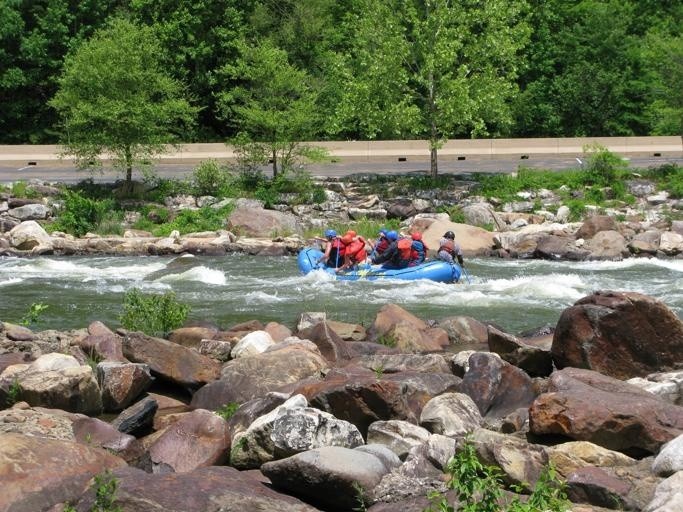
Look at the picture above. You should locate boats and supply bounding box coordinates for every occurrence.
[297,247,461,287]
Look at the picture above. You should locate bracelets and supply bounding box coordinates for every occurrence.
[317,259,320,263]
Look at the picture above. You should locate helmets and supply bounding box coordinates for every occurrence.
[380,228,397,241]
[412,232,421,240]
[325,229,336,236]
[342,231,356,242]
[443,231,455,238]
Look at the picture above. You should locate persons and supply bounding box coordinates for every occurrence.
[367,228,429,270]
[346,229,356,238]
[314,228,345,268]
[334,234,368,274]
[435,230,465,268]
